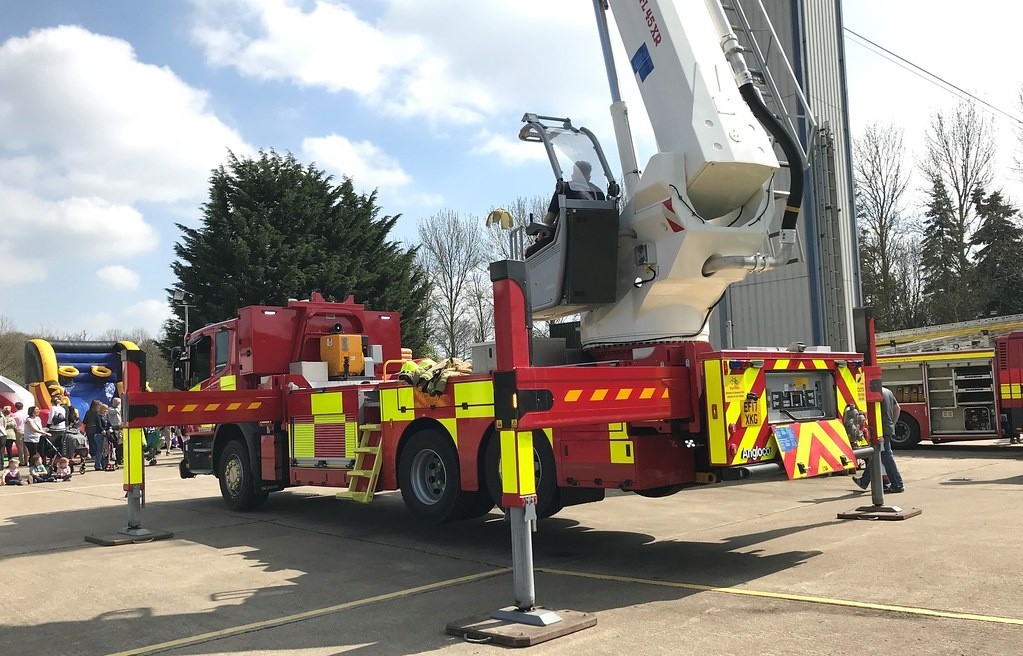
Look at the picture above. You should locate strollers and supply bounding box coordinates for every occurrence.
[44,427,90,475]
[142,426,167,466]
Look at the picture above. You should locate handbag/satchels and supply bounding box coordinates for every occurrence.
[53,406,66,425]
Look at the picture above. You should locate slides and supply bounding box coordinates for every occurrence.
[58,376,122,431]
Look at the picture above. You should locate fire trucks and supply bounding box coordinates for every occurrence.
[876,312,1023,451]
[80,0,924,647]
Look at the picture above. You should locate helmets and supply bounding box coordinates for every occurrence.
[401,361,418,373]
[420,359,436,368]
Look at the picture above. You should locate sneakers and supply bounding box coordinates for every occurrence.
[883,484,904,493]
[852,476,869,490]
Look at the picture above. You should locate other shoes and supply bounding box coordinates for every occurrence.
[46,478,55,482]
[20,481,28,485]
[166,451,170,456]
[63,477,71,482]
[95,467,104,471]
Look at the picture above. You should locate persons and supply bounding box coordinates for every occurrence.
[0,395,206,485]
[853,387,904,493]
[525,162,606,243]
